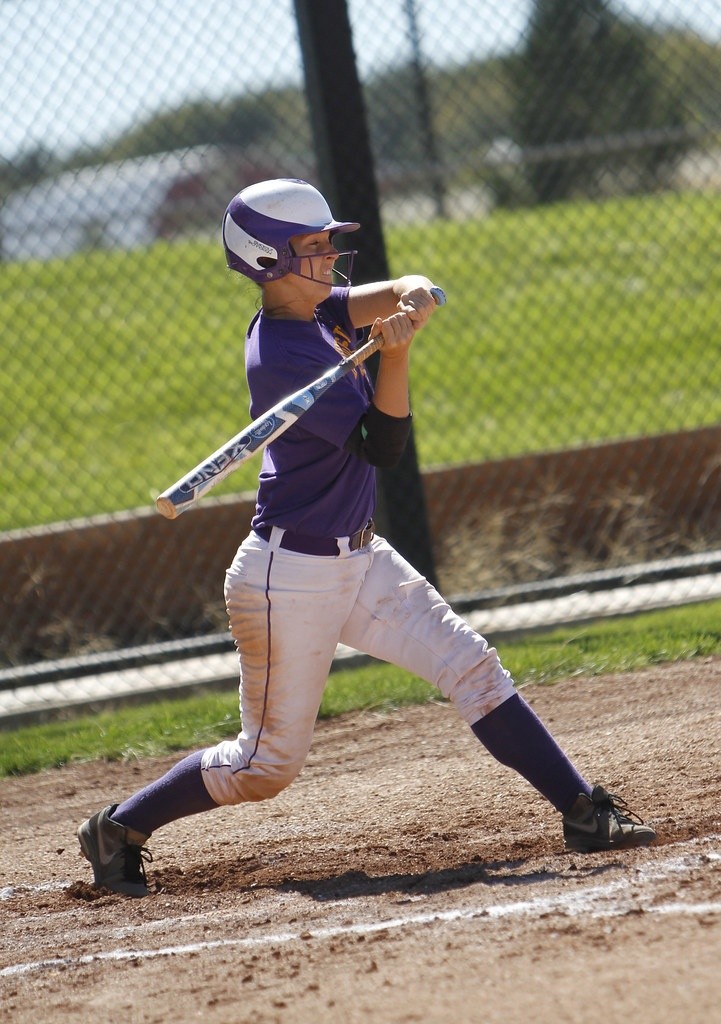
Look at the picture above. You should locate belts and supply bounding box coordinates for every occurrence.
[255,519,374,556]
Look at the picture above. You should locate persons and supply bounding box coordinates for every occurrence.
[80,177,656,893]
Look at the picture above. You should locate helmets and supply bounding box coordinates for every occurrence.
[221,179,358,288]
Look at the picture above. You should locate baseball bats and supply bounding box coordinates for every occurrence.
[152,286,448,522]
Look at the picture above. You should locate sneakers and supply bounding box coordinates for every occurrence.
[561,785,655,854]
[78,804,153,899]
